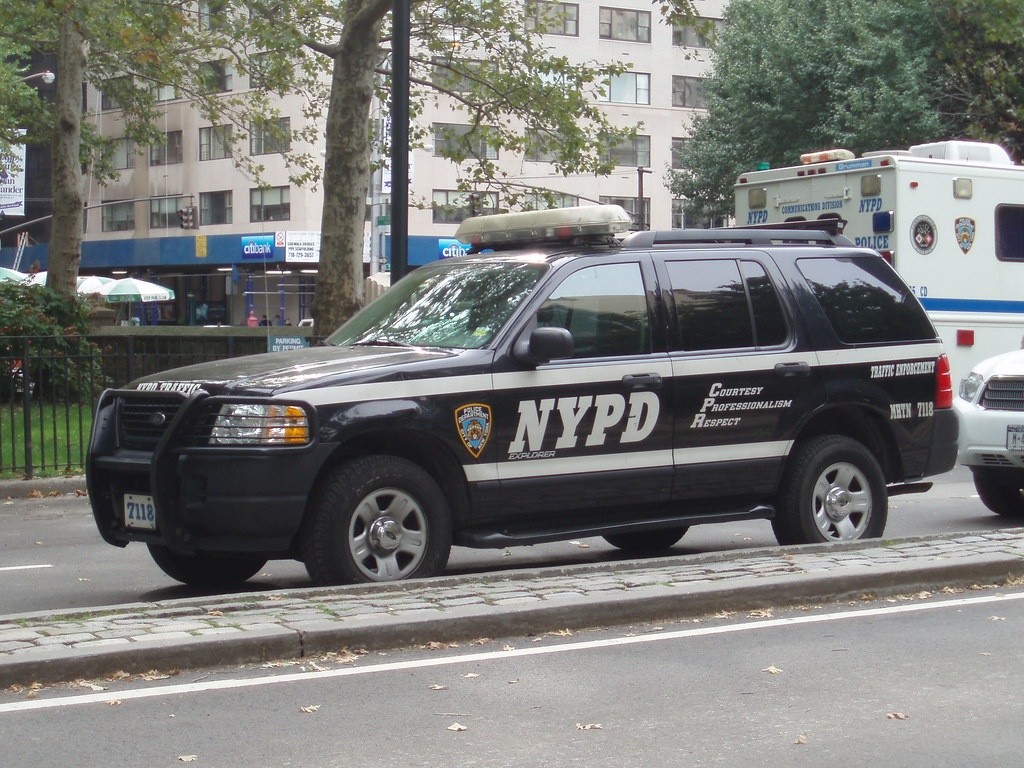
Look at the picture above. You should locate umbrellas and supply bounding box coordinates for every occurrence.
[0,267,28,282]
[17,271,46,286]
[76,275,116,297]
[86,277,175,326]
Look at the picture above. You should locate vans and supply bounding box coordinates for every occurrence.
[731,141,1024,427]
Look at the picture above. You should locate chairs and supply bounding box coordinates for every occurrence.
[567,286,646,359]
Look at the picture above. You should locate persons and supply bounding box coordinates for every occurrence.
[258,315,271,326]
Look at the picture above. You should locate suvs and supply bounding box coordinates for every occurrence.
[85,201,960,592]
